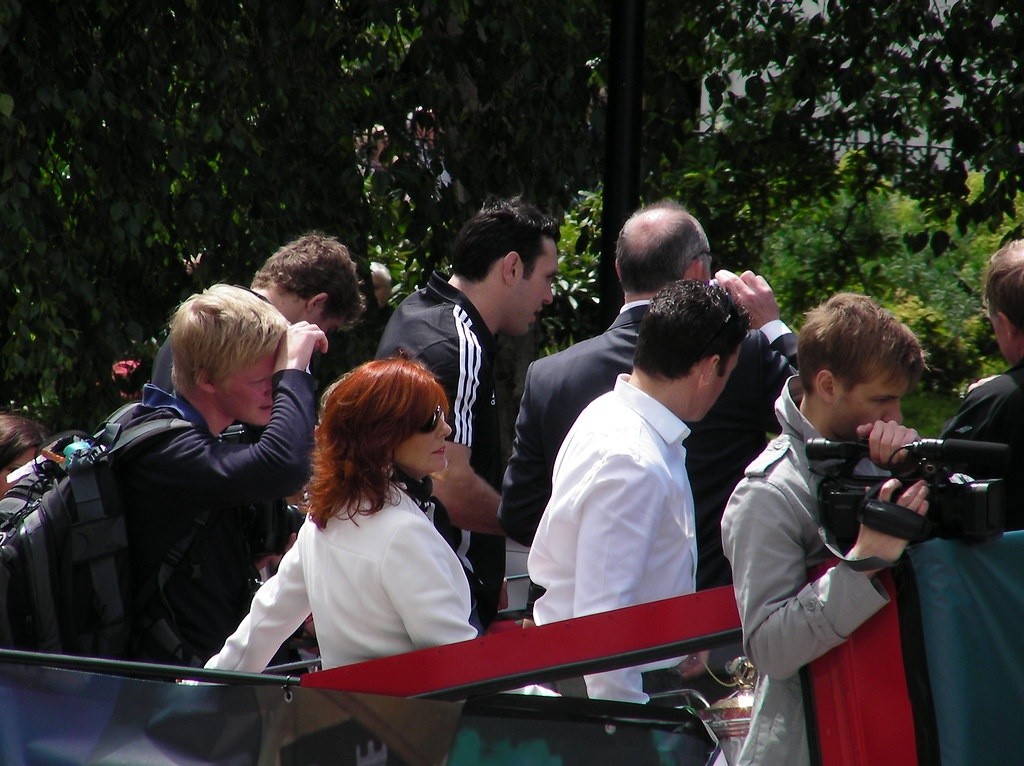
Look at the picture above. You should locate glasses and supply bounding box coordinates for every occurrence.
[417,403,444,434]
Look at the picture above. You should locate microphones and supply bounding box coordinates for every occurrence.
[906,438,1011,465]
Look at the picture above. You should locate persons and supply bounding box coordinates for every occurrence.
[525,280,748,701]
[497,205,798,701]
[721,294,931,766]
[96,285,328,684]
[151,235,389,428]
[0,413,45,501]
[172,357,476,695]
[352,106,457,227]
[936,238,1024,530]
[373,198,558,628]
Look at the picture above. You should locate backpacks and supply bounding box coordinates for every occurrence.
[0,418,211,718]
